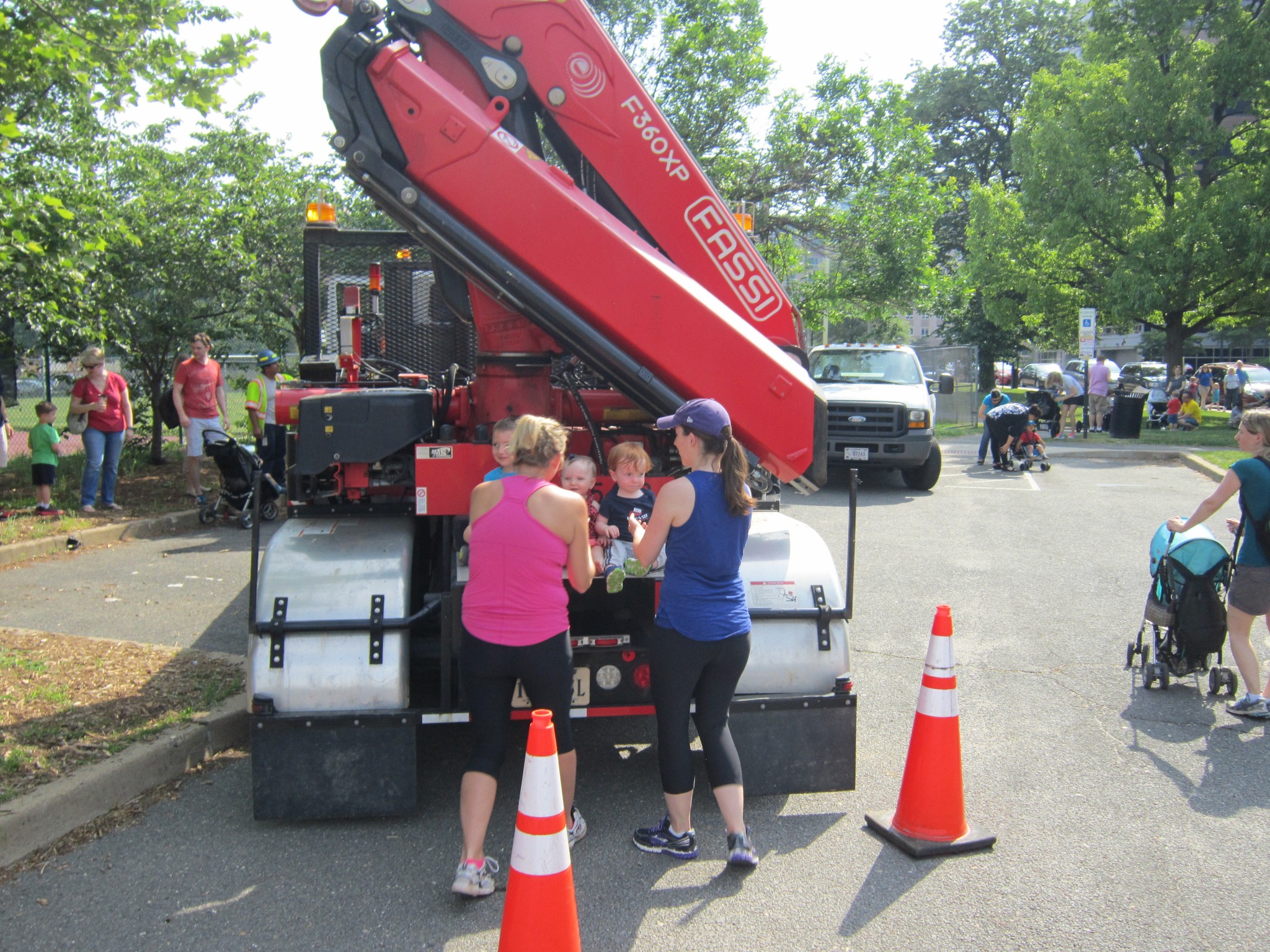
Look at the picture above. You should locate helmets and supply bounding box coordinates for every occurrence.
[255,350,281,366]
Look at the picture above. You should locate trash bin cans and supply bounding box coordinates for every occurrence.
[1109,389,1150,439]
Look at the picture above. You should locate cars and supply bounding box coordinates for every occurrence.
[1017,363,1064,390]
[920,361,968,382]
[993,362,1014,385]
[967,360,980,383]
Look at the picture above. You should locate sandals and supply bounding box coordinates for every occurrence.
[1000,465,1017,472]
[992,461,1000,470]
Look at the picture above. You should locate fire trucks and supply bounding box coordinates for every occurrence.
[201,0,860,822]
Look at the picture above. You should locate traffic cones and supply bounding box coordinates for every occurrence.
[858,606,1001,861]
[500,707,586,952]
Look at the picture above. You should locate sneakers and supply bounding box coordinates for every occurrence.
[450,857,501,896]
[633,813,698,858]
[1225,692,1270,718]
[726,829,759,865]
[566,808,587,850]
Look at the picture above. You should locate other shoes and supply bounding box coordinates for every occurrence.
[1054,433,1066,439]
[274,484,286,494]
[1042,456,1049,461]
[200,485,213,492]
[1200,407,1208,411]
[1030,456,1037,462]
[1089,428,1096,432]
[1068,434,1074,439]
[606,568,624,593]
[623,557,653,577]
[194,495,208,507]
[1096,429,1106,434]
[977,459,984,464]
[0,503,122,521]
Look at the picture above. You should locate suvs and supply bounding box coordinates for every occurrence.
[1117,361,1168,400]
[808,343,954,492]
[1189,362,1270,409]
[1062,358,1121,395]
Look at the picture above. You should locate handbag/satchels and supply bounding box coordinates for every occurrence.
[65,402,90,434]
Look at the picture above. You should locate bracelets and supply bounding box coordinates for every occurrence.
[127,427,134,430]
[4,419,9,423]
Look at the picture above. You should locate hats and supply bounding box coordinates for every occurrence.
[657,398,732,441]
[1026,420,1037,426]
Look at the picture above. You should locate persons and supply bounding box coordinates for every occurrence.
[627,399,758,867]
[1044,355,1117,442]
[555,454,610,573]
[1160,359,1251,431]
[69,348,134,513]
[27,399,65,516]
[976,386,1048,472]
[0,381,14,519]
[484,415,523,483]
[1166,402,1270,722]
[245,349,296,492]
[594,443,666,593]
[173,331,231,505]
[452,413,596,895]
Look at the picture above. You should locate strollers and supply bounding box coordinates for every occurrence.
[1008,432,1052,471]
[199,428,282,530]
[1123,510,1247,697]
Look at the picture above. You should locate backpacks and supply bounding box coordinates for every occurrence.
[1164,556,1231,655]
[157,388,180,429]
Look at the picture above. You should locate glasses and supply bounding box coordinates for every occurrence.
[82,364,98,370]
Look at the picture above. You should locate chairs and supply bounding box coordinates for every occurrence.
[880,365,907,381]
[818,365,842,380]
